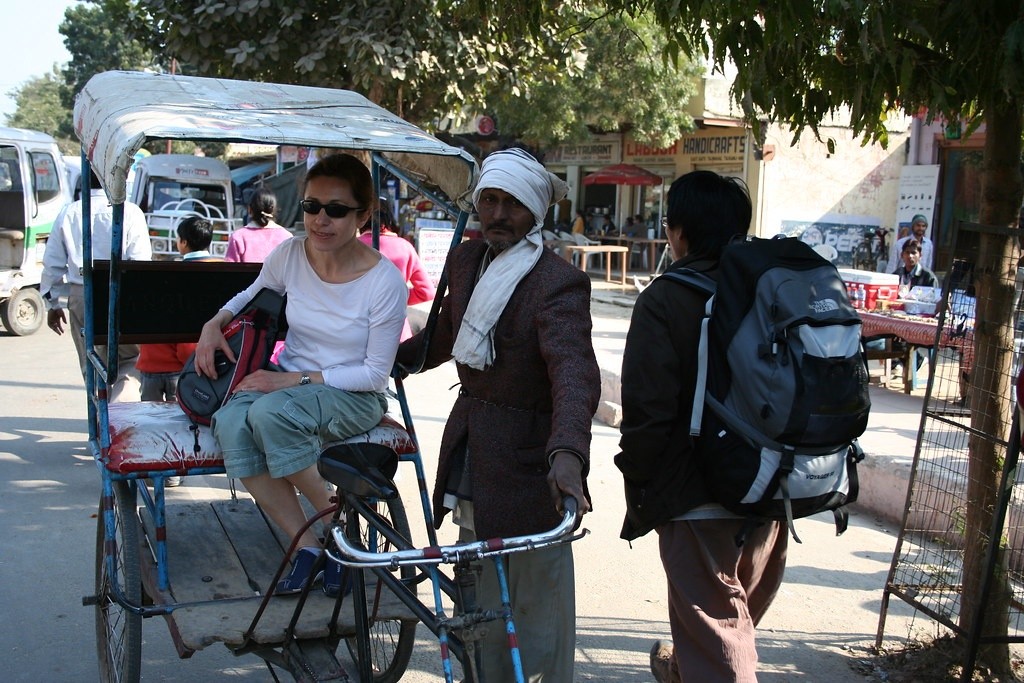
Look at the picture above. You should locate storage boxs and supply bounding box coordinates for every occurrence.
[839,269,899,311]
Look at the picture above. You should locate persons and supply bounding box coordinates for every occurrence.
[886,214,934,274]
[139,218,228,486]
[602,215,615,235]
[391,147,601,683]
[617,215,647,250]
[195,152,408,597]
[544,193,593,237]
[613,171,788,683]
[224,189,293,262]
[40,172,152,401]
[890,239,940,376]
[357,195,435,344]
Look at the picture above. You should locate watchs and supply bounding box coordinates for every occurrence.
[301,371,311,385]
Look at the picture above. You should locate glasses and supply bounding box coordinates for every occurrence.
[661,214,674,226]
[299,200,363,218]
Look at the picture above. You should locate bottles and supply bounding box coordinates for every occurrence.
[845,282,851,304]
[851,283,859,308]
[858,284,866,308]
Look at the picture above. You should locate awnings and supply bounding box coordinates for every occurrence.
[231,159,275,186]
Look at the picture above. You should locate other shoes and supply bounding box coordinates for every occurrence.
[917,352,927,373]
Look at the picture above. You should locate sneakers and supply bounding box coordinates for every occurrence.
[323,546,352,598]
[275,547,325,593]
[650,639,676,683]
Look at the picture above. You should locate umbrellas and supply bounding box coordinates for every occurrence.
[584,161,662,235]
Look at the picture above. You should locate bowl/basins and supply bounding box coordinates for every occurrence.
[602,208,611,214]
[594,208,602,213]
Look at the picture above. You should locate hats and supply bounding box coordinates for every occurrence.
[912,215,928,228]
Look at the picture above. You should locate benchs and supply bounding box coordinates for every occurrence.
[0,190,57,238]
[90,259,417,474]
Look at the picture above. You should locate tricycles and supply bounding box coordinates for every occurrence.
[67,70,593,683]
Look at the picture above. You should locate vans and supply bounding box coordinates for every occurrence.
[0,125,74,337]
[129,150,236,261]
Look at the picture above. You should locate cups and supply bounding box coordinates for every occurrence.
[601,230,604,236]
[647,229,655,239]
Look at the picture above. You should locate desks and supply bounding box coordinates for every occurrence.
[589,236,668,273]
[853,309,976,395]
[566,245,628,284]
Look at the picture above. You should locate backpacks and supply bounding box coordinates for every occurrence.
[653,234,871,546]
[176,287,281,454]
